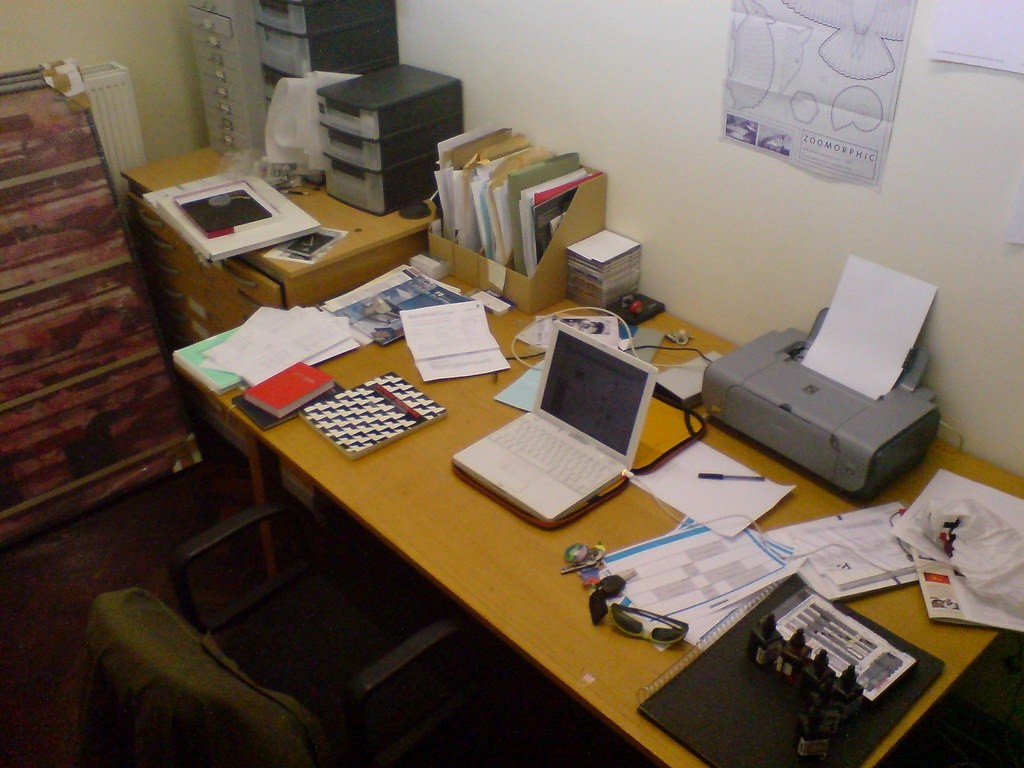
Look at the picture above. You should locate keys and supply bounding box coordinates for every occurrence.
[560,545,640,596]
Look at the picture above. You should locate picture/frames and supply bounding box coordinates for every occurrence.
[0,59,204,547]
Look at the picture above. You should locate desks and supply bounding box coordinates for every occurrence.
[172,256,1024,768]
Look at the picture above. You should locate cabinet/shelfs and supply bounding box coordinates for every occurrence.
[121,148,428,516]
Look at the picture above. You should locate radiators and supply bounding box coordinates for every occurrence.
[80,59,146,206]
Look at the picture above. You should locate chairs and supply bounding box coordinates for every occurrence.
[78,498,478,768]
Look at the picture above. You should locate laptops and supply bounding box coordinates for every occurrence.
[452,320,658,523]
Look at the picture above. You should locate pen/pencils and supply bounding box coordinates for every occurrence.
[697,472,765,481]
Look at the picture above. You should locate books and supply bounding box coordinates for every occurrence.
[639,573,946,768]
[171,262,475,431]
[299,371,446,459]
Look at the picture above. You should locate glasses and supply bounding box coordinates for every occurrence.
[611,603,689,644]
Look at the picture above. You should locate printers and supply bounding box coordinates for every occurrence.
[702,308,941,509]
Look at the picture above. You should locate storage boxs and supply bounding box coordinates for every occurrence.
[434,169,607,315]
[190,0,465,216]
